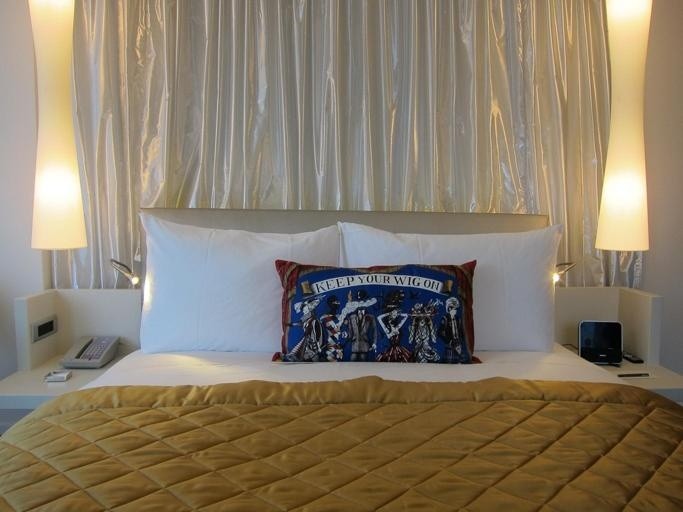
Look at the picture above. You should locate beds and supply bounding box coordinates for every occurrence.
[0,208,683,512]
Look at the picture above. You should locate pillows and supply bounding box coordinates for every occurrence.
[337,221,562,352]
[271,259,484,365]
[137,213,340,355]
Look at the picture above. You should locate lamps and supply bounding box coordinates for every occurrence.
[553,262,576,282]
[595,0,653,251]
[110,259,141,289]
[28,0,87,250]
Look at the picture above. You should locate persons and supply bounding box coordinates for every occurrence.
[287,287,464,363]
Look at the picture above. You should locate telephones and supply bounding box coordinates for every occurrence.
[63,335,120,369]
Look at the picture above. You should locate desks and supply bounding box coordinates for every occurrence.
[555,286,683,406]
[0,289,141,436]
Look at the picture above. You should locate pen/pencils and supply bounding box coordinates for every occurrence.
[618,373,649,377]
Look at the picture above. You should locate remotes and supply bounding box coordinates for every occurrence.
[623,350,644,363]
